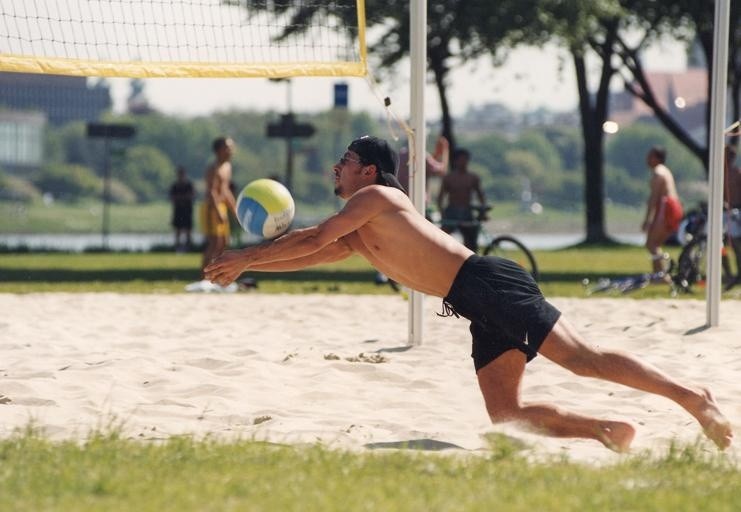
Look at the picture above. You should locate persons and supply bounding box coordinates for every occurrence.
[642,145,683,286]
[168,165,197,249]
[435,148,489,252]
[377,133,451,287]
[203,135,736,456]
[201,133,240,284]
[720,146,741,293]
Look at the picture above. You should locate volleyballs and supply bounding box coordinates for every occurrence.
[236,179,295,239]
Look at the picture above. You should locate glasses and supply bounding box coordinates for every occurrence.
[337,154,360,168]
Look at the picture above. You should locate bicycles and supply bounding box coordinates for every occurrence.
[385,203,543,301]
[577,252,676,296]
[671,204,741,297]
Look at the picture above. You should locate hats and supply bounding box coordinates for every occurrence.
[347,133,407,192]
